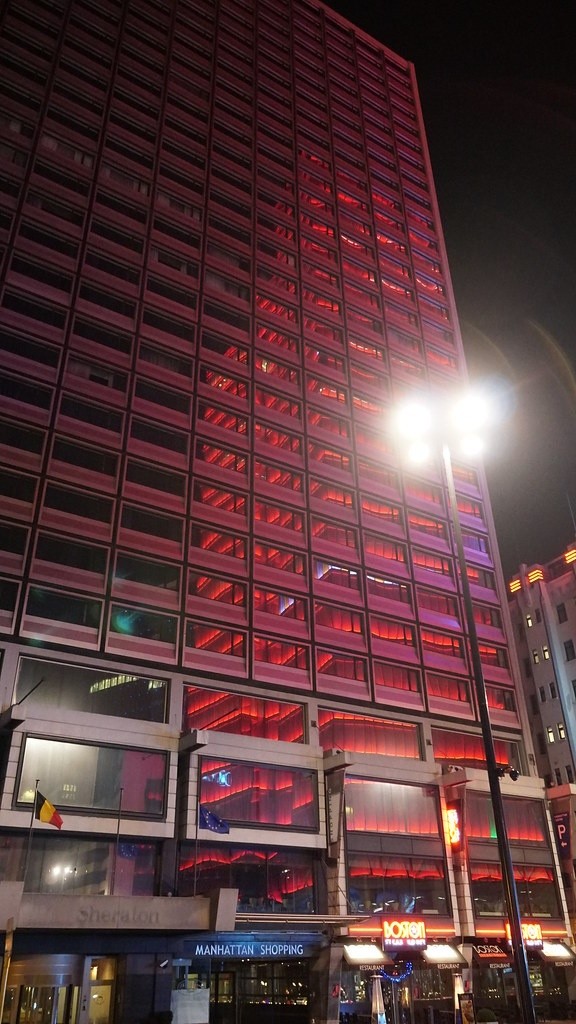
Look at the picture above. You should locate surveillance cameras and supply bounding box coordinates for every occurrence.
[509,771,520,781]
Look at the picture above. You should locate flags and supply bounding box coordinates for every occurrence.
[116,841,138,859]
[32,783,64,830]
[196,801,230,834]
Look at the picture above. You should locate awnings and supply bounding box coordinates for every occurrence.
[536,939,576,966]
[472,943,514,964]
[420,943,468,969]
[344,942,396,971]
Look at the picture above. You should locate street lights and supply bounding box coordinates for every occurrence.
[386,382,539,1023]
